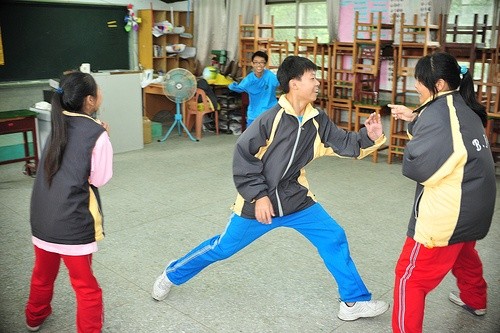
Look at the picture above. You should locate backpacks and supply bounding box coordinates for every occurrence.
[195,77,217,110]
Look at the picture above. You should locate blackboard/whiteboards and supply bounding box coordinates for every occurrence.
[0,0,140,86]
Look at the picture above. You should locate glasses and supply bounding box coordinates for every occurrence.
[252,61,267,66]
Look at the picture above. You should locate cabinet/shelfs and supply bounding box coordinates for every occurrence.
[138,9,195,76]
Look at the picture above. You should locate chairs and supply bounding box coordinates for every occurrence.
[182,88,219,141]
[238,10,500,164]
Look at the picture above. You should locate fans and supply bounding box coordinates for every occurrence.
[157,68,200,143]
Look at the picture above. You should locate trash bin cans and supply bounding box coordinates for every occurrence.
[142,117,152,144]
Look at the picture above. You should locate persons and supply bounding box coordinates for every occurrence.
[387,52,497,333]
[25,72,113,333]
[152,55,390,322]
[228,51,280,128]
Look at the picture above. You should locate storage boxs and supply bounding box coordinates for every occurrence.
[211,50,227,65]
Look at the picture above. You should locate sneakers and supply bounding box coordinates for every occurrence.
[338,298,390,320]
[449,291,486,316]
[152,271,173,301]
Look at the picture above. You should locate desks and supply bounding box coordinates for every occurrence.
[142,75,232,139]
[0,108,41,176]
